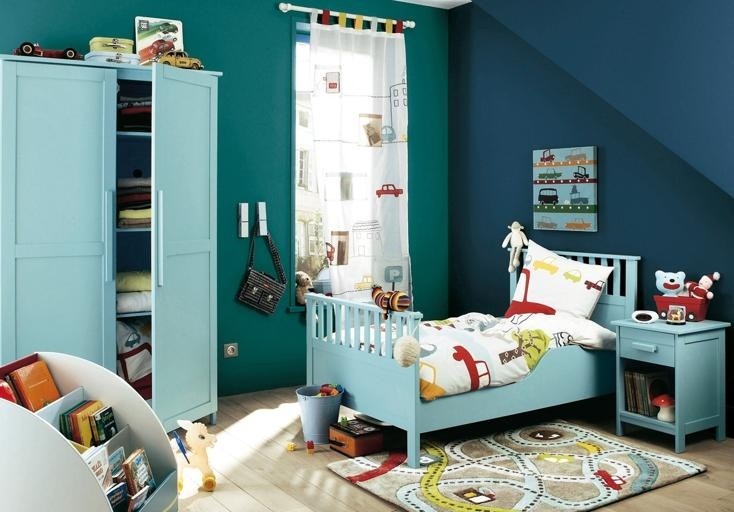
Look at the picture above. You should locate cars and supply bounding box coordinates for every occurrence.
[147,20,178,55]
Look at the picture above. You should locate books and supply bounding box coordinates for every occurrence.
[7,361,157,512]
[624,369,675,417]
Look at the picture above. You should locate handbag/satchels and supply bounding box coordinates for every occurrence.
[237,267,284,313]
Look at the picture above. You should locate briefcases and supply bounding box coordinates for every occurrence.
[84,37,140,65]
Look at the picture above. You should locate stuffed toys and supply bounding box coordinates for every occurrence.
[296,270,317,305]
[369,283,411,319]
[502,221,529,273]
[685,271,720,300]
[655,270,686,297]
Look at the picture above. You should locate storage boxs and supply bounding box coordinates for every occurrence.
[327,418,384,458]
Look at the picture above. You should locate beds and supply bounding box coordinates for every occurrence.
[303,247,641,468]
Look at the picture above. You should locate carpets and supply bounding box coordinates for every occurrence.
[325,416,708,512]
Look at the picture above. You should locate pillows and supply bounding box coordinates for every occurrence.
[504,239,615,319]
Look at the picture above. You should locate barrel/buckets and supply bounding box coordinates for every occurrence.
[297,385,346,444]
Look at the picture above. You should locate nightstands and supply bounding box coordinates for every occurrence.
[609,318,731,453]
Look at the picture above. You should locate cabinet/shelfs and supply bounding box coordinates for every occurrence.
[0,352,177,512]
[0,54,223,435]
